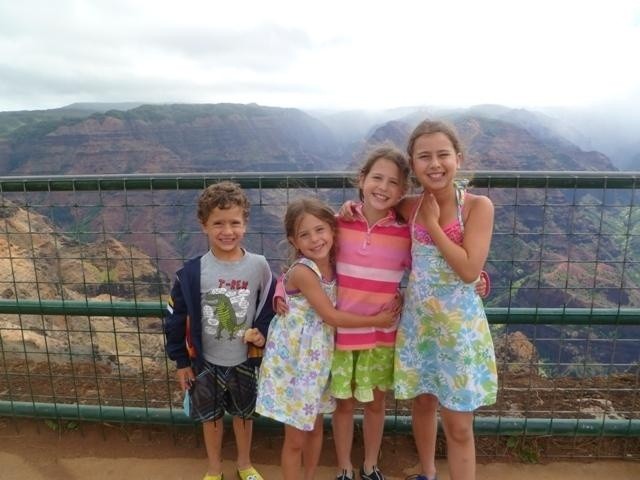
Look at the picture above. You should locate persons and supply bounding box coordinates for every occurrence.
[161,178,279,480]
[338,117,501,480]
[270,142,492,480]
[251,195,404,480]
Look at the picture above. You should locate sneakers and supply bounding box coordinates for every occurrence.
[204,471,224,480]
[358,464,385,480]
[236,465,264,480]
[334,468,355,480]
